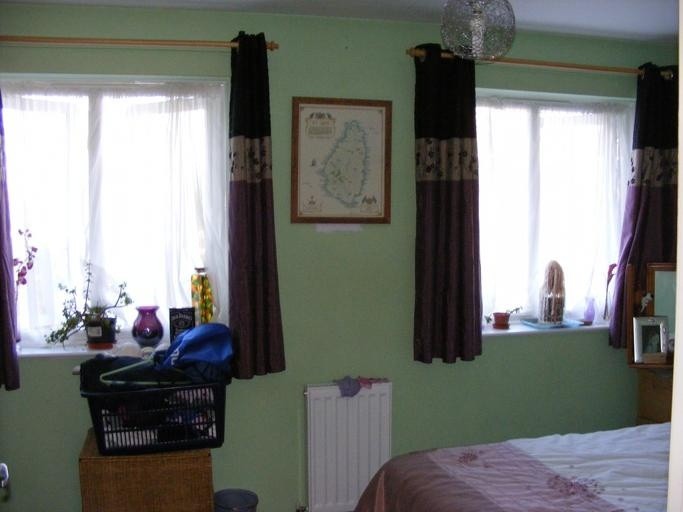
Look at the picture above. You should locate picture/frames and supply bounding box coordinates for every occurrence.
[647,262,676,362]
[290,95,393,224]
[633,315,669,364]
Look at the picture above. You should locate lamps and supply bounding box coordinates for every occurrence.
[438,0,516,60]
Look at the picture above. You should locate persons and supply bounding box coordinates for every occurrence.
[643,328,660,353]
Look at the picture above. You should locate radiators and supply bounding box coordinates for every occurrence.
[304,379,392,511]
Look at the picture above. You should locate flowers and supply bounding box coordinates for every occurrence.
[10,228,38,342]
[44,259,132,350]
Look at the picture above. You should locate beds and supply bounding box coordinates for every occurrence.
[353,421,671,512]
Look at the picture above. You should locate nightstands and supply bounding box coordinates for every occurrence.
[629,364,672,426]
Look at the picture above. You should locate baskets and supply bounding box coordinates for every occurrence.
[79,361,234,458]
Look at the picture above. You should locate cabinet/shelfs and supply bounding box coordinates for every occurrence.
[78,426,215,512]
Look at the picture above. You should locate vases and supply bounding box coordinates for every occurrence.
[131,306,164,349]
[82,318,117,343]
[14,321,22,354]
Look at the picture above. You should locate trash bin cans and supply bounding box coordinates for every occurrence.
[214,488,260,512]
[0,463,10,503]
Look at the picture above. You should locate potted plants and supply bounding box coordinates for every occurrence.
[484,305,522,329]
